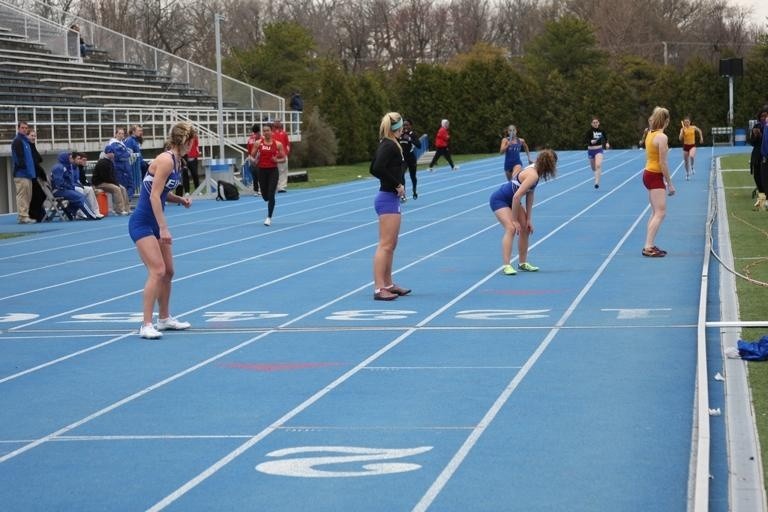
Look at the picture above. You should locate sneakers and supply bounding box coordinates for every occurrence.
[516,263,540,272]
[111,207,128,216]
[17,216,37,224]
[138,321,163,340]
[502,264,517,276]
[278,189,287,193]
[156,316,192,331]
[125,210,134,215]
[263,217,272,226]
[373,287,399,301]
[690,167,696,175]
[686,174,691,181]
[95,212,105,219]
[76,210,88,219]
[642,245,667,258]
[254,191,262,197]
[385,284,412,297]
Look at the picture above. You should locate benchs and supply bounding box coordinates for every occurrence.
[0,26,269,140]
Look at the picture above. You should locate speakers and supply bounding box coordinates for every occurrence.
[719,58,744,76]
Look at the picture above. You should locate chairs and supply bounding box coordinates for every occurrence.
[36,176,79,222]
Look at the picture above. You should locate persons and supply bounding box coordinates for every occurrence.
[638,114,654,146]
[501,125,535,182]
[748,113,768,210]
[128,122,196,339]
[427,119,459,173]
[10,119,291,227]
[639,106,676,257]
[678,117,703,180]
[490,148,558,274]
[397,118,419,201]
[585,118,609,191]
[370,112,412,301]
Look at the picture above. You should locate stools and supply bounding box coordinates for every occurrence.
[95,188,109,217]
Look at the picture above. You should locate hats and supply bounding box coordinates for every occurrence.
[104,145,115,153]
